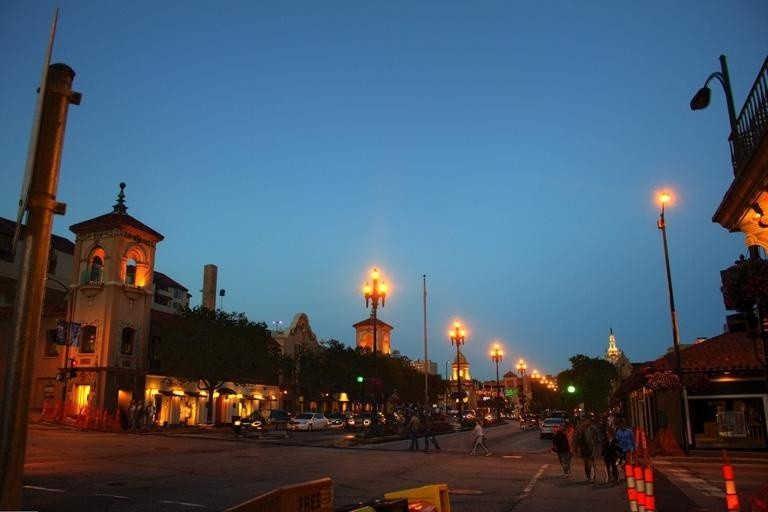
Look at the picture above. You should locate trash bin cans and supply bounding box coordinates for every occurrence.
[233,415,240,429]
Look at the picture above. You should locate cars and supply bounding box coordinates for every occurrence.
[445,408,571,441]
[291,413,329,432]
[326,414,346,431]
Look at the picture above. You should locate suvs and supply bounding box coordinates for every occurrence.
[348,411,386,432]
[233,410,293,441]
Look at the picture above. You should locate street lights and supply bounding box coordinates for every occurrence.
[272,319,282,333]
[653,189,692,458]
[686,50,766,366]
[44,276,74,402]
[362,269,387,437]
[448,319,465,424]
[516,359,558,419]
[491,343,503,424]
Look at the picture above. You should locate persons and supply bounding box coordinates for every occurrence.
[518,407,636,485]
[406,412,492,457]
[128,400,157,433]
